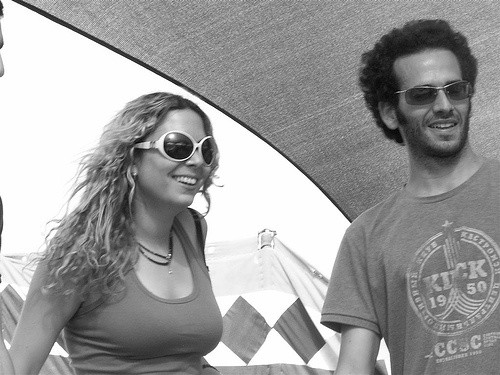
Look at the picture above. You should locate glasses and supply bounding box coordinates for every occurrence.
[133,132,215,166]
[394,81,472,107]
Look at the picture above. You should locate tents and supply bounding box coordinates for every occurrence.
[0,228,391,375]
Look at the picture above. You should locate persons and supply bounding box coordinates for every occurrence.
[320,19,500,375]
[0,0,14,375]
[9,92,223,375]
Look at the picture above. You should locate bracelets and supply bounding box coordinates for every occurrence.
[201,365,220,372]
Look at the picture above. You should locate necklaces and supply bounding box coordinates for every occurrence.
[135,229,174,274]
